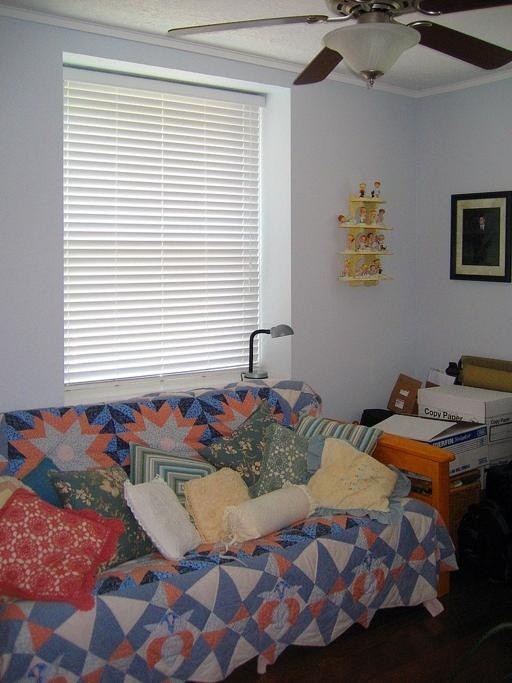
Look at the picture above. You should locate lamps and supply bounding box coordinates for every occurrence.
[321,9,421,88]
[243,325,294,379]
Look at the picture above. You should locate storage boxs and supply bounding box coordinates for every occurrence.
[457,356,512,392]
[418,385,512,462]
[387,374,438,416]
[373,412,488,481]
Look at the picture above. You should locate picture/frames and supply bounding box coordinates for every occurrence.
[450,191,511,283]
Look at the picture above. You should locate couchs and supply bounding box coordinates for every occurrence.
[0,380,454,683]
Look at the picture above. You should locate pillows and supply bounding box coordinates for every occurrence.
[227,480,313,538]
[48,465,158,574]
[123,474,205,563]
[250,424,310,500]
[129,441,217,528]
[295,415,384,458]
[0,487,125,612]
[198,399,285,497]
[184,467,251,545]
[303,435,412,525]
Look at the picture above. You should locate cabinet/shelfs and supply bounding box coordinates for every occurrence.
[336,197,395,287]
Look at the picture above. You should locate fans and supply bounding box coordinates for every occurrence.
[166,1,509,85]
